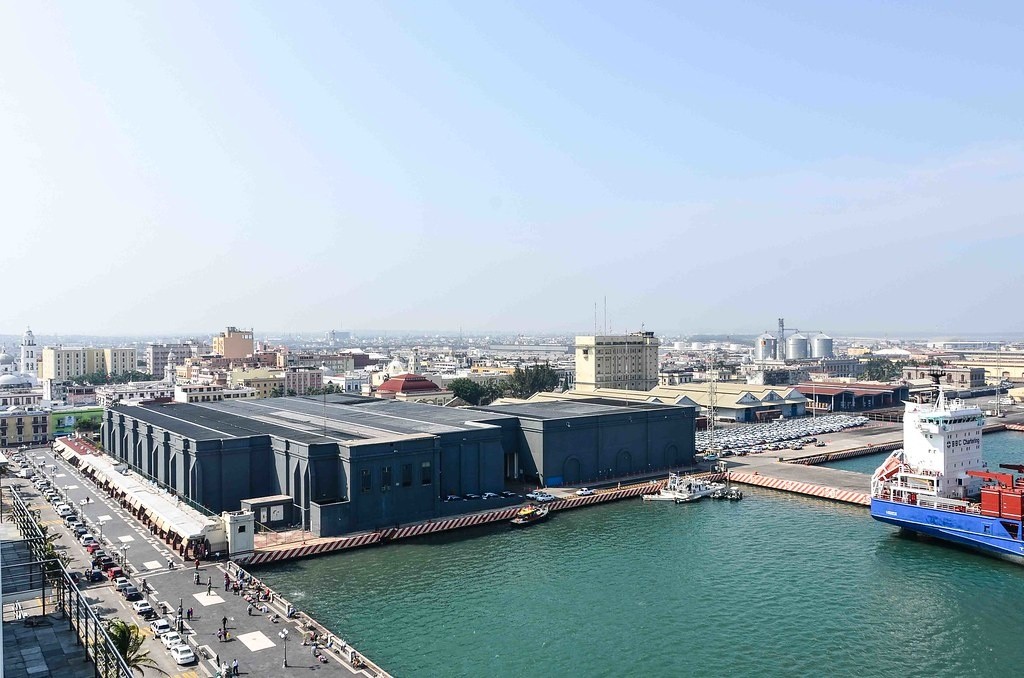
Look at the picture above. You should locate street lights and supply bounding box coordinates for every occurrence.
[62,484,69,504]
[96,521,106,541]
[39,462,45,475]
[49,472,56,487]
[120,543,131,567]
[29,452,36,466]
[278,628,288,668]
[78,502,86,520]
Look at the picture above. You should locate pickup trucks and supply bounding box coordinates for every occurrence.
[64,570,80,588]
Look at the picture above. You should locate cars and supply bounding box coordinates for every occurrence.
[161,632,182,651]
[695,415,869,457]
[171,644,195,665]
[526,490,540,501]
[695,414,870,462]
[133,599,152,615]
[89,570,103,583]
[576,488,593,496]
[447,491,516,501]
[13,452,140,601]
[537,494,555,503]
[151,618,171,640]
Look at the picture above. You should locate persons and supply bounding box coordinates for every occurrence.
[178,605,194,621]
[224,568,298,618]
[167,546,220,569]
[39,450,90,505]
[216,615,231,642]
[300,616,362,668]
[193,571,212,596]
[221,659,240,677]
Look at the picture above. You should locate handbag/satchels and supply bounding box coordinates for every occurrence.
[218,634,222,636]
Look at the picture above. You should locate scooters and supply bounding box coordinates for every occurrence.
[144,611,160,622]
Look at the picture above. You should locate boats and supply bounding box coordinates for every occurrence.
[641,473,726,504]
[728,487,743,502]
[869,448,1024,565]
[508,505,548,528]
